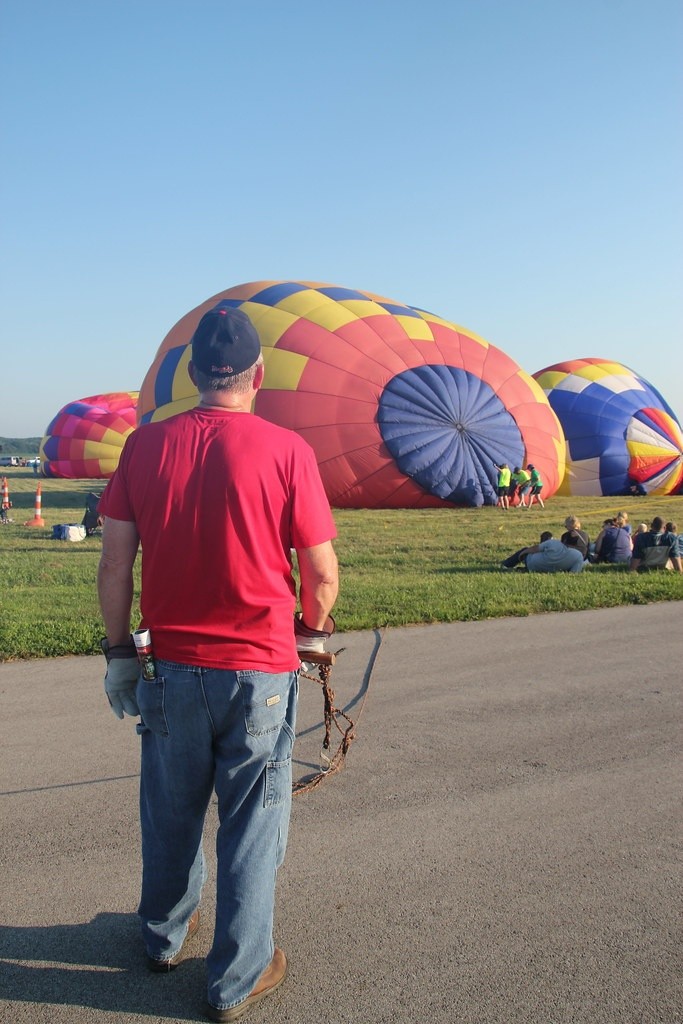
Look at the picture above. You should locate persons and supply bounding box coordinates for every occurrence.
[98,305,338,1024]
[501,510,683,575]
[493,463,544,510]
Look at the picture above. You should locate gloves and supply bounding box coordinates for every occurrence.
[100,638,142,719]
[294,611,336,673]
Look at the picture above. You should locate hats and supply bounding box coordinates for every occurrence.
[193,306,260,377]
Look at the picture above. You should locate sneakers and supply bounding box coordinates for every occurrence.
[209,946,288,1022]
[146,909,200,972]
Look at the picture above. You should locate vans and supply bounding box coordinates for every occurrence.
[0,455,22,467]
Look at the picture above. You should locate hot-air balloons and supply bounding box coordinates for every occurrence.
[136,280,568,510]
[529,356,683,497]
[38,390,139,479]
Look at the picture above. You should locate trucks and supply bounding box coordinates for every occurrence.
[25,456,41,468]
